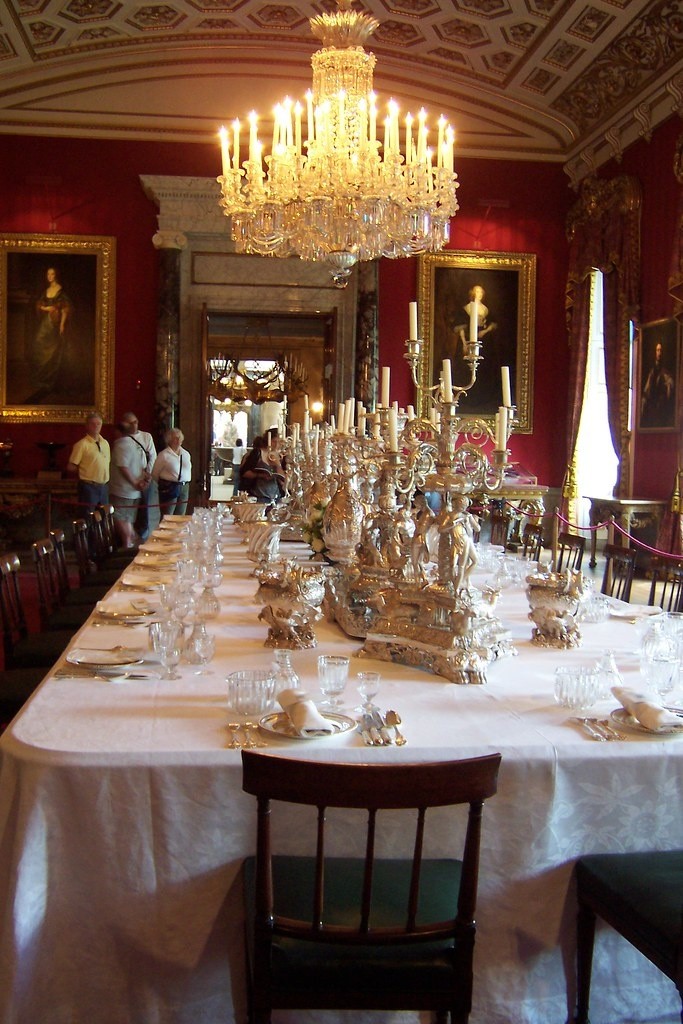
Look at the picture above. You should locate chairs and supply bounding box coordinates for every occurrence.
[557,531,587,573]
[568,849,683,1024]
[601,544,638,603]
[488,513,514,552]
[521,523,544,561]
[229,745,502,1024]
[0,503,137,727]
[645,553,683,612]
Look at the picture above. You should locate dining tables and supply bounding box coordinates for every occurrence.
[0,504,683,1024]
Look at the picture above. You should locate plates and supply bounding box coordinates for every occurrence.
[610,706,683,734]
[123,514,188,589]
[95,599,156,619]
[66,647,144,667]
[258,711,358,740]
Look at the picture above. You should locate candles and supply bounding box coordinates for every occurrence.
[267,298,519,471]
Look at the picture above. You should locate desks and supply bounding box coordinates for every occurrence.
[457,482,550,554]
[580,494,669,573]
[0,478,81,551]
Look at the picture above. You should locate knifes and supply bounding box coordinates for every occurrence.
[56,669,149,677]
[354,710,392,747]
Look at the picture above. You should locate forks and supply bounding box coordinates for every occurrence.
[226,721,267,749]
[570,716,624,742]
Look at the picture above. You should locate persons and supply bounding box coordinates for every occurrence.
[239,428,292,502]
[232,438,246,496]
[67,414,111,512]
[108,412,158,549]
[410,494,481,600]
[151,428,192,522]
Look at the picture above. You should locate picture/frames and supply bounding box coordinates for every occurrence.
[416,249,540,437]
[635,314,681,433]
[0,232,120,426]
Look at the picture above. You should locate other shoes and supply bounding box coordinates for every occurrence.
[118,541,133,553]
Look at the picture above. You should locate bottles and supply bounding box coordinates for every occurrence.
[270,649,301,711]
[638,619,674,683]
[593,650,624,698]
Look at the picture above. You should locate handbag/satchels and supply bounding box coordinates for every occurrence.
[238,449,260,492]
[158,483,179,503]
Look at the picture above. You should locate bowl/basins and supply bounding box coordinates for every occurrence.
[228,670,277,714]
[555,664,598,709]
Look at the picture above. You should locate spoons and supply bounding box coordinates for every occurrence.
[58,672,132,682]
[385,710,407,746]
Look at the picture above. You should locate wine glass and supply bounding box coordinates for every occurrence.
[471,539,610,623]
[660,610,683,662]
[318,656,349,714]
[354,672,379,712]
[151,499,288,682]
[650,657,680,708]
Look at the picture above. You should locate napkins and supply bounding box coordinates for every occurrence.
[277,688,335,737]
[612,683,683,732]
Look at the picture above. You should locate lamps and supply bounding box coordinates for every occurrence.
[217,0,462,287]
[209,317,306,406]
